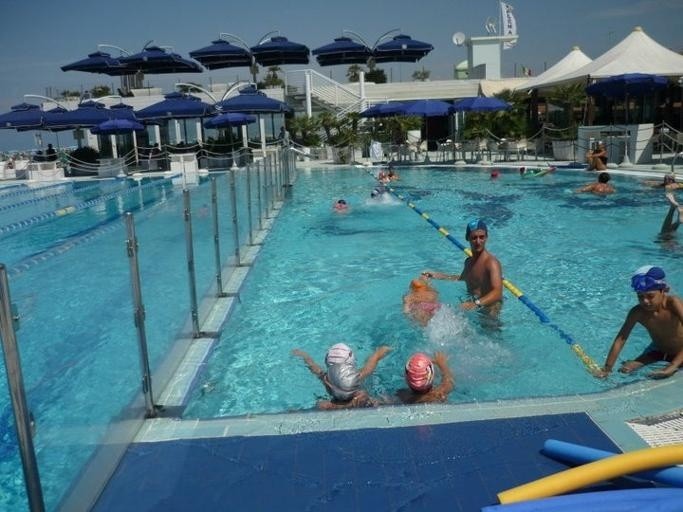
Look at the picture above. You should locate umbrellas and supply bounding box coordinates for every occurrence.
[1,87,292,164]
[514,25,683,125]
[355,96,510,142]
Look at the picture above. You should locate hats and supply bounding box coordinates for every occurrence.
[630,265,670,292]
[323,343,360,400]
[466,218,487,236]
[404,352,434,392]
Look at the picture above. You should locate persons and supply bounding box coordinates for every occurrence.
[577,172,683,378]
[577,141,615,171]
[333,198,349,213]
[520,166,556,179]
[491,169,500,178]
[291,343,454,409]
[403,218,503,328]
[379,169,400,182]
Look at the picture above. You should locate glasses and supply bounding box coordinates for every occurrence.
[631,275,666,290]
